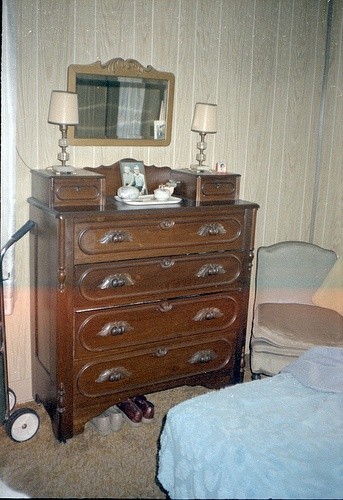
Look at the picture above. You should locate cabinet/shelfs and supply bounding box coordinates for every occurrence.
[27,158,260,443]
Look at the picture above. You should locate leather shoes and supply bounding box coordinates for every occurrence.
[115,398,142,423]
[133,397,154,420]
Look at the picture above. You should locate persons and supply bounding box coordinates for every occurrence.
[133,166,147,195]
[123,166,136,187]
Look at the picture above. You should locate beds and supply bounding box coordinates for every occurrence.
[158,347,343,500]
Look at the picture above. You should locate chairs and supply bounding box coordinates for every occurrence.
[251,242,343,380]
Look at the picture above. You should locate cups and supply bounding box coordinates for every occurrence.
[163,187,174,195]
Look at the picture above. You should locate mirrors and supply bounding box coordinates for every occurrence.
[67,57,175,146]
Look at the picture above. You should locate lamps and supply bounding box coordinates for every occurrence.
[191,103,218,173]
[48,90,79,176]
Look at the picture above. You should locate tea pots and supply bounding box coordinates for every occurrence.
[154,184,170,200]
